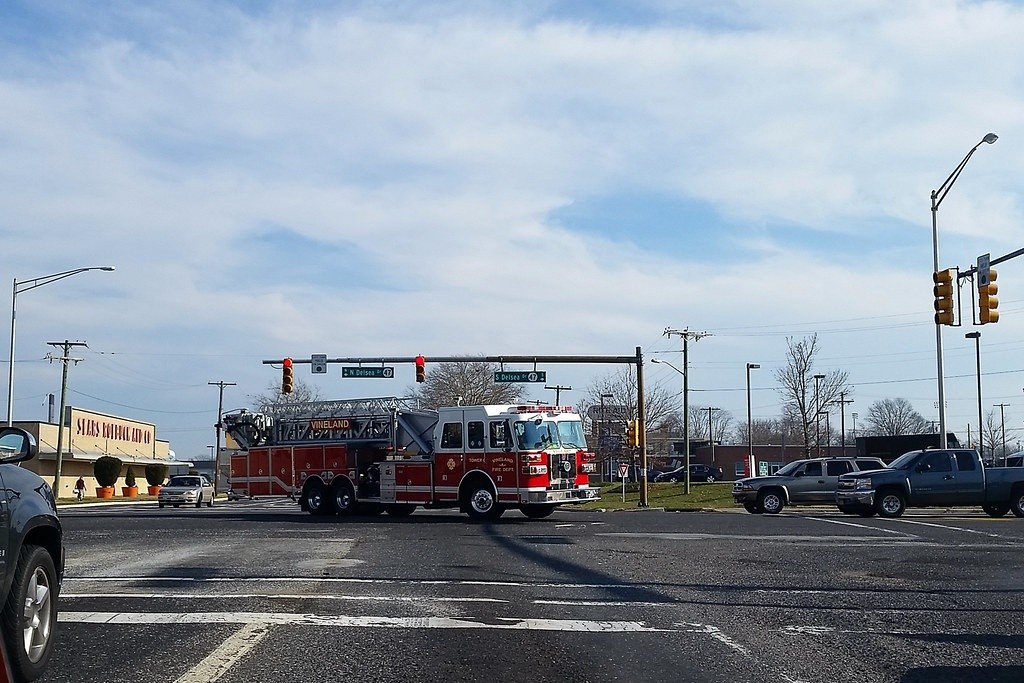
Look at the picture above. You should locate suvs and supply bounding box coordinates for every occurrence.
[732,456,888,514]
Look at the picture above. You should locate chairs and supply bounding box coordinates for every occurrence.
[189,480,196,486]
[176,481,184,485]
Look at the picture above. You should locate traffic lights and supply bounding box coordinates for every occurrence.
[933,270,954,326]
[978,269,999,323]
[415,356,425,382]
[282,357,294,394]
[625,420,639,447]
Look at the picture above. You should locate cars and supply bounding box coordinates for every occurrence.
[158,476,216,508]
[653,464,723,483]
[0,425,66,683]
[228,487,250,502]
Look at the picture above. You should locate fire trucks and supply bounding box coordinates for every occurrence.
[214,394,602,524]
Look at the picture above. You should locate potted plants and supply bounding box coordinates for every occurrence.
[145,463,167,496]
[122,466,138,496]
[94,456,123,499]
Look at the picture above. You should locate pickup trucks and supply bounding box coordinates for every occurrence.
[834,446,1024,519]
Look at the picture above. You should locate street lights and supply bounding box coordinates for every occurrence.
[651,358,689,496]
[965,330,984,459]
[818,411,830,456]
[931,131,999,450]
[206,445,214,461]
[600,393,613,483]
[814,374,825,457]
[7,265,116,429]
[746,362,760,478]
